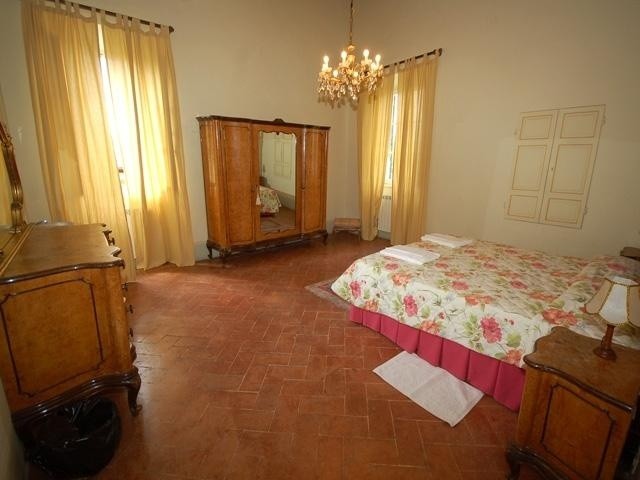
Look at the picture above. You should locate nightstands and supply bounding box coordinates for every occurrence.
[504,326,640,480]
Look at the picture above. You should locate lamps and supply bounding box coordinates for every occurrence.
[314,0,384,103]
[585,276,640,362]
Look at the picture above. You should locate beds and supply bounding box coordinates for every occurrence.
[259,185,282,217]
[330,232,640,412]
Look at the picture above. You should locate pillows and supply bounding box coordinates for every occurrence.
[537,256,640,348]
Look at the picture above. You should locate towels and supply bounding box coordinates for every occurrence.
[379,233,475,265]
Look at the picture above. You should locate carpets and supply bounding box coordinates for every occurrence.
[304,274,351,318]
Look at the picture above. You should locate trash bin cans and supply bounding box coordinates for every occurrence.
[22,397,119,480]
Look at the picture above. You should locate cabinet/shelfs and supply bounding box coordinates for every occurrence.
[1,222,144,464]
[195,115,331,264]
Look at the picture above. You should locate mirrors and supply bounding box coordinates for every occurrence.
[1,120,26,277]
[257,129,298,235]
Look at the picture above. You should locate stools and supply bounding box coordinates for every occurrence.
[333,217,361,246]
